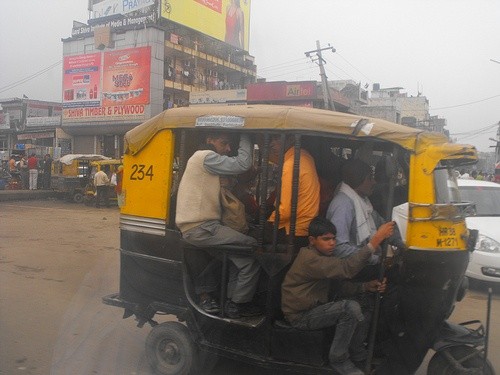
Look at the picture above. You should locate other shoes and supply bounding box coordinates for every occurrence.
[224,302,263,318]
[353,361,376,375]
[329,359,365,375]
[196,292,222,313]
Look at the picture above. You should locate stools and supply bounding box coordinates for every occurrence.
[270,319,334,366]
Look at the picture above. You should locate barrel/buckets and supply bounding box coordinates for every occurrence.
[73,177,82,189]
[64,178,73,190]
[0,177,7,189]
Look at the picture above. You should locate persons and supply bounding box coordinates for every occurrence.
[10,155,27,189]
[27,153,39,191]
[175,134,266,319]
[225,0,244,50]
[231,134,398,359]
[281,217,396,375]
[94,167,112,209]
[455,167,493,181]
[40,154,51,189]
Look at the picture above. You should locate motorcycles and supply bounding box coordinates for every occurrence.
[50,154,122,205]
[103,104,494,373]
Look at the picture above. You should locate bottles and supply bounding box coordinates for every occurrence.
[77,88,86,100]
[90,89,93,99]
[94,84,97,98]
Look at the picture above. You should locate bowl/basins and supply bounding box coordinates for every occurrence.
[10,183,21,191]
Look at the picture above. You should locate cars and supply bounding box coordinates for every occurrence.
[391,176,500,283]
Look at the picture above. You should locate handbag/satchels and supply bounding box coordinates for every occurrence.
[221,186,249,234]
[111,171,118,186]
